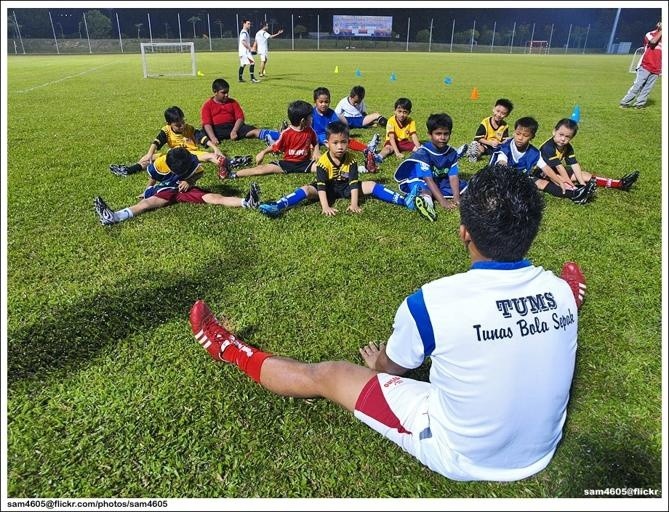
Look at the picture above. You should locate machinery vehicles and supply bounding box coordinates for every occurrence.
[333,21,355,36]
[358,23,368,34]
[371,23,391,36]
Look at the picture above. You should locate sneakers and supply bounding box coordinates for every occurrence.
[364,134,383,173]
[403,184,437,222]
[571,176,596,204]
[244,182,279,219]
[457,141,478,162]
[265,134,283,156]
[94,196,115,227]
[620,171,639,190]
[218,156,253,179]
[190,300,236,362]
[110,164,130,176]
[560,262,586,307]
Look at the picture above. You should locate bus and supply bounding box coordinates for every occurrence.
[526,40,548,48]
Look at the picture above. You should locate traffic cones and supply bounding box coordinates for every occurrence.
[471,86,479,99]
[443,76,453,84]
[390,71,397,80]
[334,65,340,73]
[355,67,361,76]
[571,104,581,123]
[197,70,205,77]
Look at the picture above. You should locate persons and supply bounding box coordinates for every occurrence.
[228,100,381,179]
[538,119,640,192]
[251,22,284,78]
[188,165,584,482]
[257,122,421,218]
[335,86,388,130]
[408,114,470,222]
[194,80,288,147]
[238,20,258,83]
[311,88,380,156]
[108,106,253,177]
[489,118,596,205]
[618,18,663,108]
[364,98,468,163]
[466,98,514,165]
[92,147,261,226]
